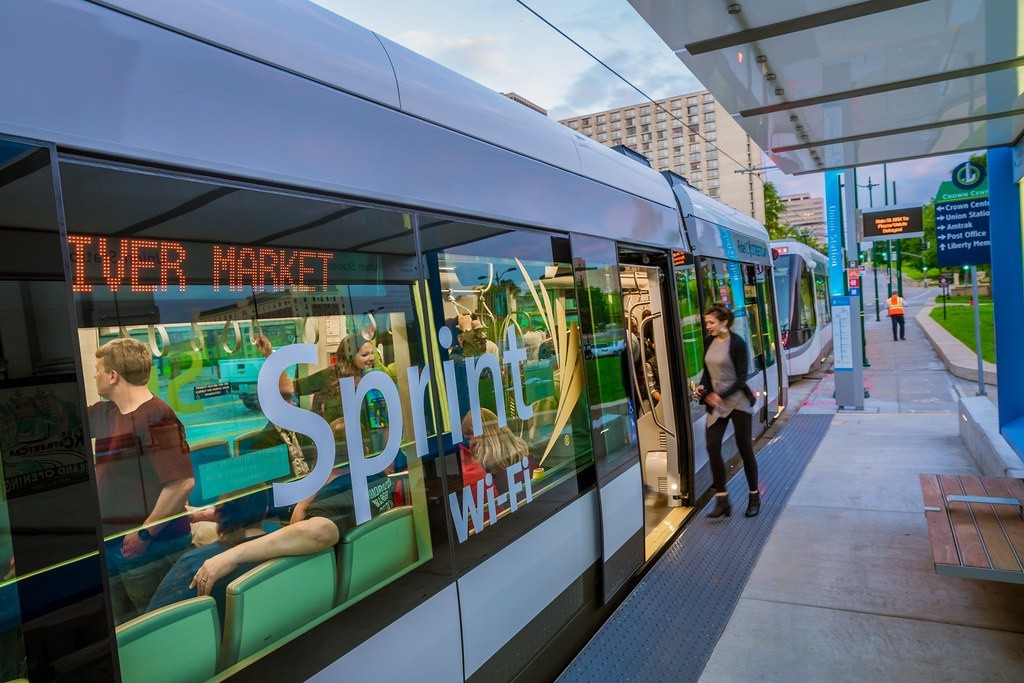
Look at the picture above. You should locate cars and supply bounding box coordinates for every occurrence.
[589,342,612,358]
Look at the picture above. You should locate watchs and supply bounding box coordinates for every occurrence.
[137,529,155,541]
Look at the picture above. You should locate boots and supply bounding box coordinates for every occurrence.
[745,491,761,516]
[705,494,731,517]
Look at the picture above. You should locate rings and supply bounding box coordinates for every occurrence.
[693,391,696,395]
[202,578,207,582]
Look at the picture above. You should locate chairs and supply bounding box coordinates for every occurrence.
[114,596,225,683]
[224,543,339,669]
[337,504,417,603]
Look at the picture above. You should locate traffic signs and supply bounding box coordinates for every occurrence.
[934,197,991,268]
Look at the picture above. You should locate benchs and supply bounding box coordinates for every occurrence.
[918,472,1024,586]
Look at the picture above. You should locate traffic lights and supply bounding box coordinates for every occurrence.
[860,254,864,259]
[923,267,927,271]
[883,253,887,257]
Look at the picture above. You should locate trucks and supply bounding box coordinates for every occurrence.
[217,339,297,409]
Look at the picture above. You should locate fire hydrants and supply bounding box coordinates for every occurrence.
[968,298,973,306]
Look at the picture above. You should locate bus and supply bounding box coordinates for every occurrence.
[770,237,833,381]
[1,0,787,683]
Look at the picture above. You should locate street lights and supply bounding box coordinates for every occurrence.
[841,177,881,322]
[477,266,518,313]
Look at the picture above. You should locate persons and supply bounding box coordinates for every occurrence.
[87,309,661,629]
[885,291,908,342]
[692,302,761,518]
